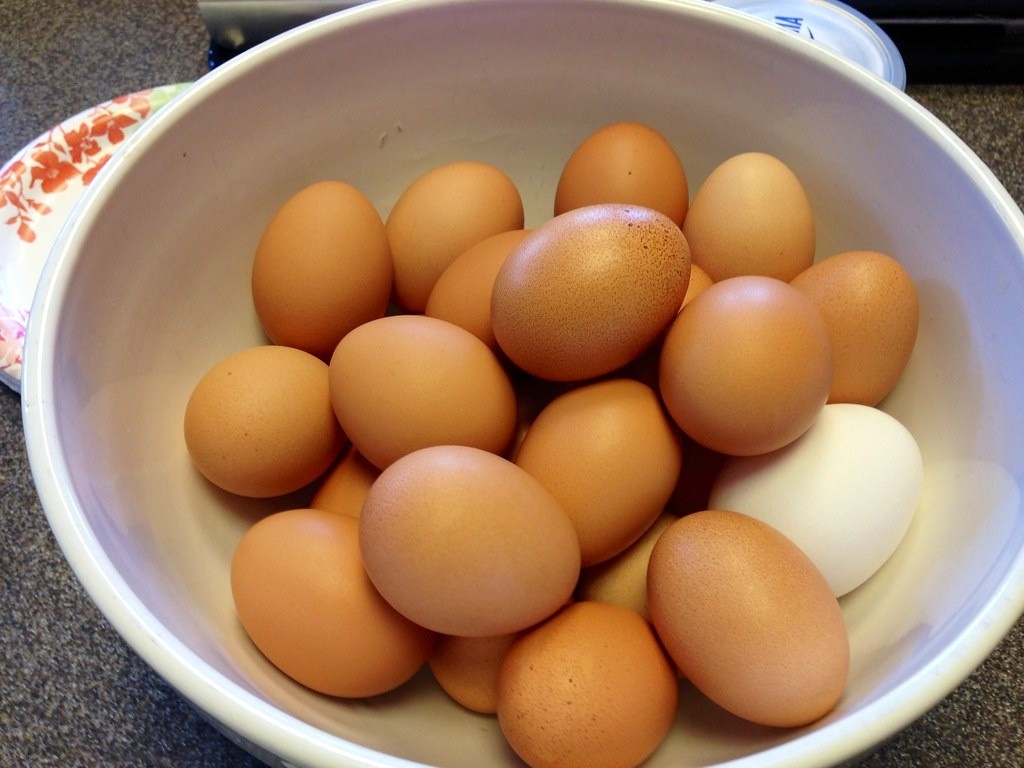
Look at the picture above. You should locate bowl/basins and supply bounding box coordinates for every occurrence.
[18,0,1023,768]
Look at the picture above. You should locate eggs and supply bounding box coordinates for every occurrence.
[185,123,925,768]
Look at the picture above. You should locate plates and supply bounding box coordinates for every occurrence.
[0,82,200,395]
